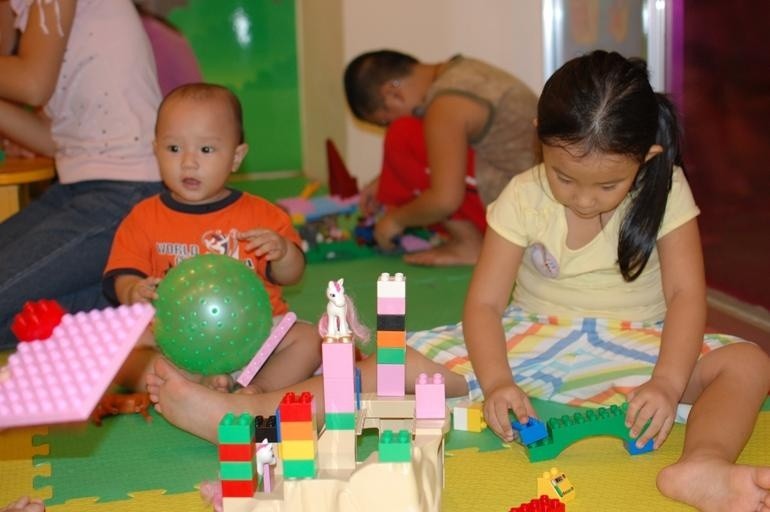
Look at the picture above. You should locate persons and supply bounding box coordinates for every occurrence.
[0,0,171,351]
[340,50,548,265]
[131,3,207,102]
[147,48,770,511]
[99,82,323,401]
